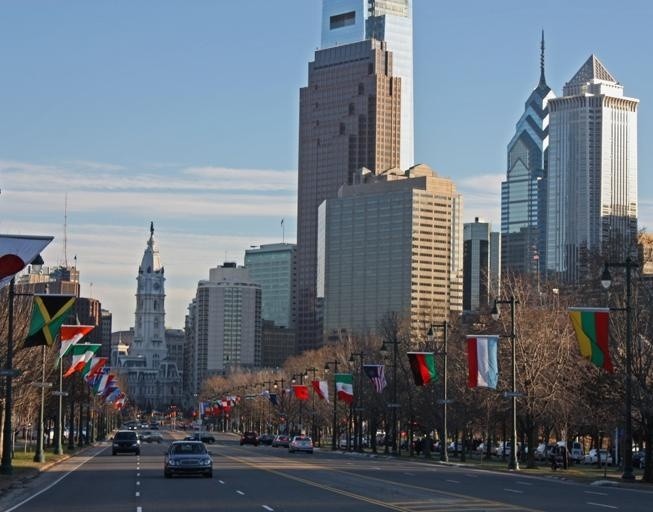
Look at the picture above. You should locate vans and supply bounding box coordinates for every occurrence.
[66,427,86,438]
[556,441,585,464]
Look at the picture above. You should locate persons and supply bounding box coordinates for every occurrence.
[413,434,564,464]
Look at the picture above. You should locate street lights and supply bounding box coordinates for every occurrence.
[302,366,320,447]
[599,258,635,479]
[325,359,340,451]
[349,351,364,453]
[32,312,82,462]
[425,320,452,463]
[262,379,273,434]
[207,382,264,436]
[1,254,44,474]
[273,378,285,434]
[192,393,197,420]
[290,372,303,436]
[491,295,521,471]
[379,337,400,455]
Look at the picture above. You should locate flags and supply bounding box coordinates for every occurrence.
[567,308,617,376]
[0,234,55,290]
[406,351,439,388]
[362,364,389,394]
[195,361,355,420]
[21,294,129,418]
[464,334,501,391]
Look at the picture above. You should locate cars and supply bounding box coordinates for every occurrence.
[341,435,367,448]
[287,436,313,454]
[629,451,644,466]
[141,423,148,429]
[584,454,592,464]
[374,431,386,446]
[271,434,290,448]
[43,426,68,441]
[638,454,645,469]
[136,430,162,443]
[400,435,554,461]
[184,433,214,444]
[587,448,612,465]
[262,435,274,445]
[128,424,136,429]
[163,439,212,478]
[178,421,199,430]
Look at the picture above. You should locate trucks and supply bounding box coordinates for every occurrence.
[149,422,159,429]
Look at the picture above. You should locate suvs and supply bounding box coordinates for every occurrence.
[110,428,140,455]
[238,431,258,447]
